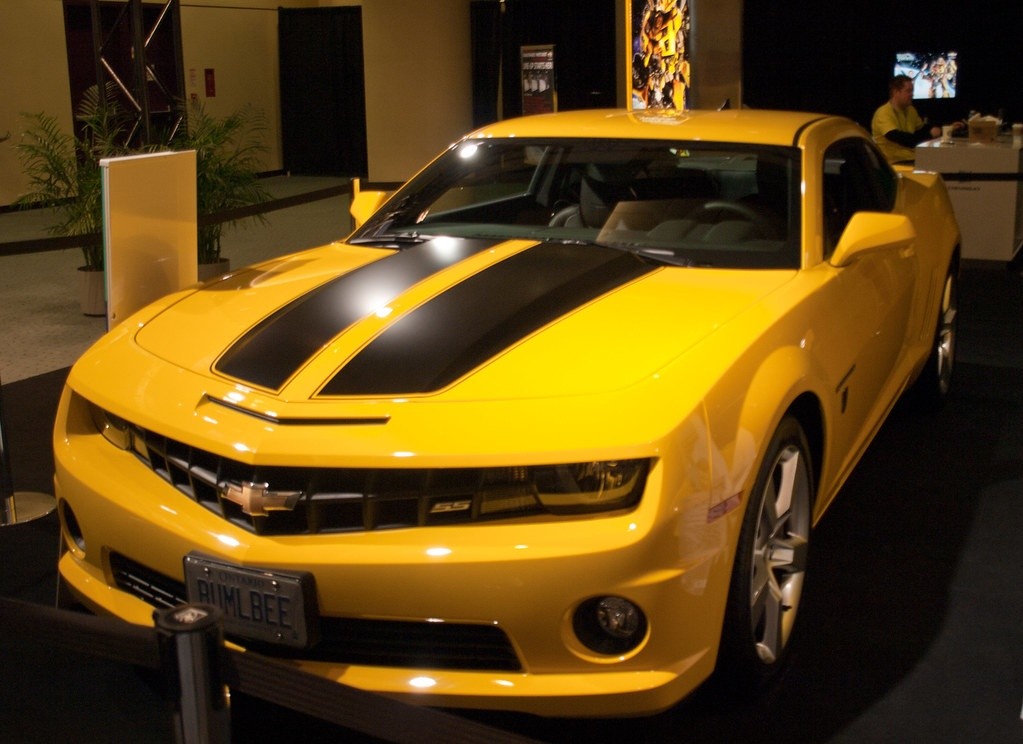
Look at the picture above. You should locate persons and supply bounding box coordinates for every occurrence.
[871,75,965,166]
[523,70,550,92]
[632,0,688,109]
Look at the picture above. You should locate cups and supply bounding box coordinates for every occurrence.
[1013,123,1023,142]
[942,125,953,142]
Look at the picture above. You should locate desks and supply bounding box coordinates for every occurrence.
[915,136,1023,261]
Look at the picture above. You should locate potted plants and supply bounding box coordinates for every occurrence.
[9,83,138,318]
[148,96,280,280]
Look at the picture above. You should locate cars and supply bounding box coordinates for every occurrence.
[46,105,964,717]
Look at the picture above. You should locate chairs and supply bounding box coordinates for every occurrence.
[549,162,637,230]
[838,154,878,203]
[728,155,790,240]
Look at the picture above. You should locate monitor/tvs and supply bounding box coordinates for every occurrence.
[893,51,958,98]
